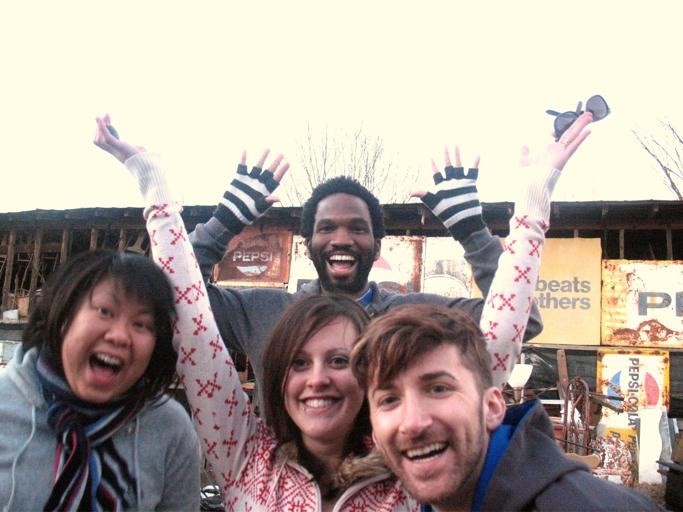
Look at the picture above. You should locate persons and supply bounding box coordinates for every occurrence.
[92,106,595,512]
[346,303,665,512]
[189,142,545,412]
[0,244,201,512]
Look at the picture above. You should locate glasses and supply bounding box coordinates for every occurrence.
[546,95,609,141]
